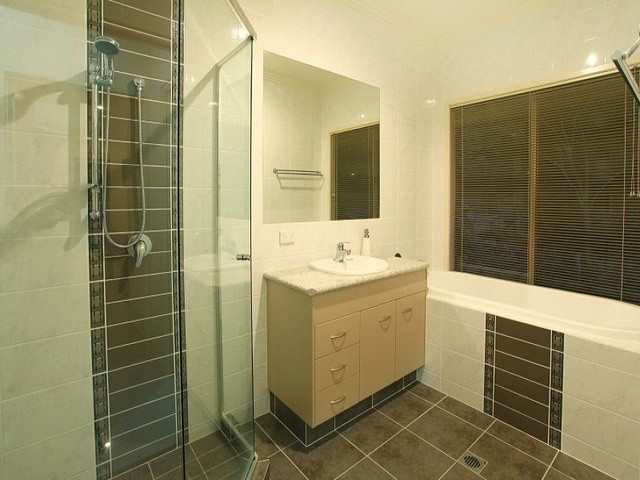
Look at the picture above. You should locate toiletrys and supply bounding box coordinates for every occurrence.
[361,229,371,255]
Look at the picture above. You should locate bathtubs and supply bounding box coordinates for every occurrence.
[427,268,639,352]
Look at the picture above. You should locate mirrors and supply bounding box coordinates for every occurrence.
[261,48,380,223]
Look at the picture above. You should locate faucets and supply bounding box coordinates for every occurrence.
[338,241,353,257]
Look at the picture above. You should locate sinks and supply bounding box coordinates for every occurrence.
[311,255,390,276]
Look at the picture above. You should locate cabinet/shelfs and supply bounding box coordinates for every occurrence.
[268,311,360,429]
[361,291,429,400]
[273,168,323,179]
